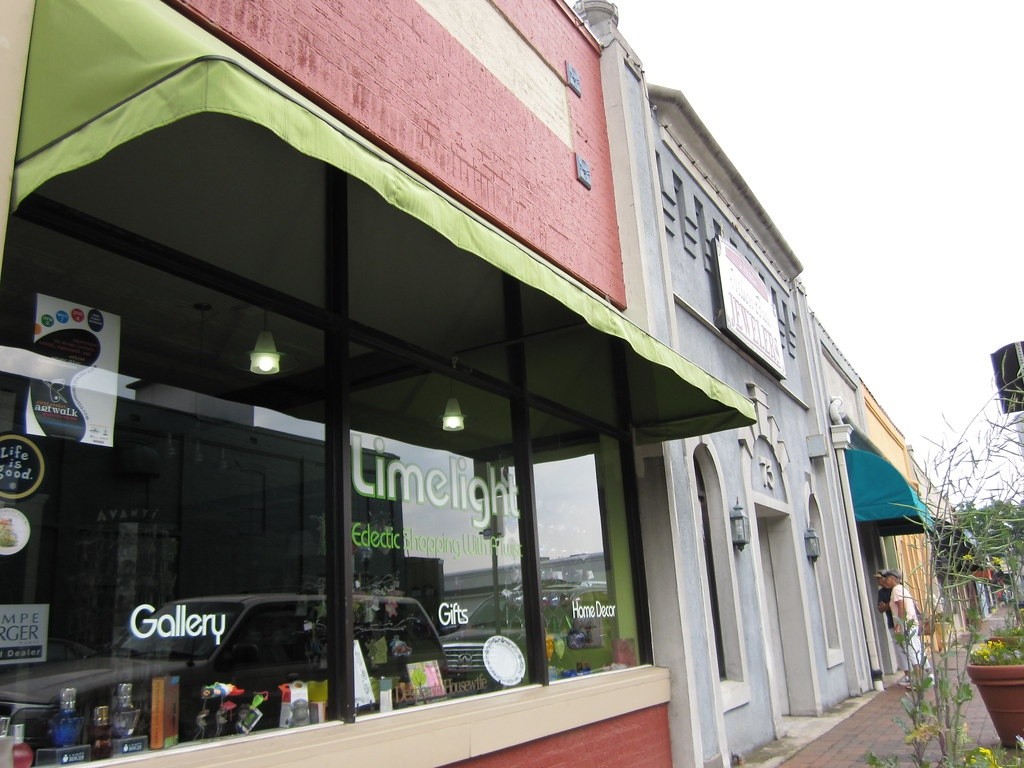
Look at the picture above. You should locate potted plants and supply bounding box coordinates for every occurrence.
[934,612,953,635]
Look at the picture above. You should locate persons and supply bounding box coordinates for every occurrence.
[874,570,913,686]
[882,569,935,690]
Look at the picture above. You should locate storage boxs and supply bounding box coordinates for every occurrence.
[380,678,393,712]
[150,676,179,749]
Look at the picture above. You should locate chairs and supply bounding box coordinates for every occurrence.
[240,616,294,661]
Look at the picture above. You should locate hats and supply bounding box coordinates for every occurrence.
[873,572,883,578]
[880,571,898,578]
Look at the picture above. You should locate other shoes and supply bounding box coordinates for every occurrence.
[899,676,915,685]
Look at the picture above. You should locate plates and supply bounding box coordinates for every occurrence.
[482,635,526,686]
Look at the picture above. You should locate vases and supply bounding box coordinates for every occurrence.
[988,635,1019,643]
[967,663,1024,748]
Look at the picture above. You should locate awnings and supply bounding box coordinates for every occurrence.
[10,1,759,450]
[844,448,997,582]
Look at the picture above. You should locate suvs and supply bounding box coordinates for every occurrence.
[0,590,451,751]
[424,574,613,684]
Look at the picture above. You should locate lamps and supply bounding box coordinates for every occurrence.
[439,379,468,431]
[249,309,285,374]
[805,522,821,563]
[165,302,229,470]
[729,496,751,552]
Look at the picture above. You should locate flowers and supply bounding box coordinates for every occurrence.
[992,608,1024,638]
[970,640,1024,666]
